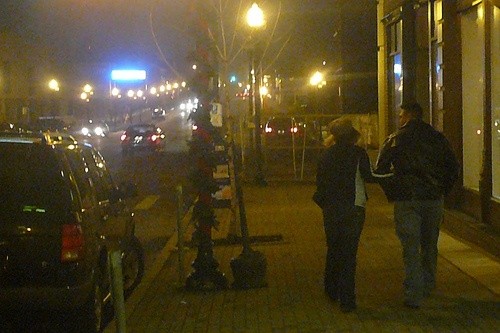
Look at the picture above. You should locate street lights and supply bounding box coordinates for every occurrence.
[244,2,268,188]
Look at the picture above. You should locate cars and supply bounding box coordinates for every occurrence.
[263,116,301,145]
[151,109,166,119]
[119,123,167,161]
[80,121,110,142]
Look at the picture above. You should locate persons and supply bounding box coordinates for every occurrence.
[373,104,458,308]
[316,118,396,313]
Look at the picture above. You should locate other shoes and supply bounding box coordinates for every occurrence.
[341,293,357,312]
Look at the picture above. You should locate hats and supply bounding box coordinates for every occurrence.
[330,117,353,137]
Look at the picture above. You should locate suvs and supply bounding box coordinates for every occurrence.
[0,130,144,333]
